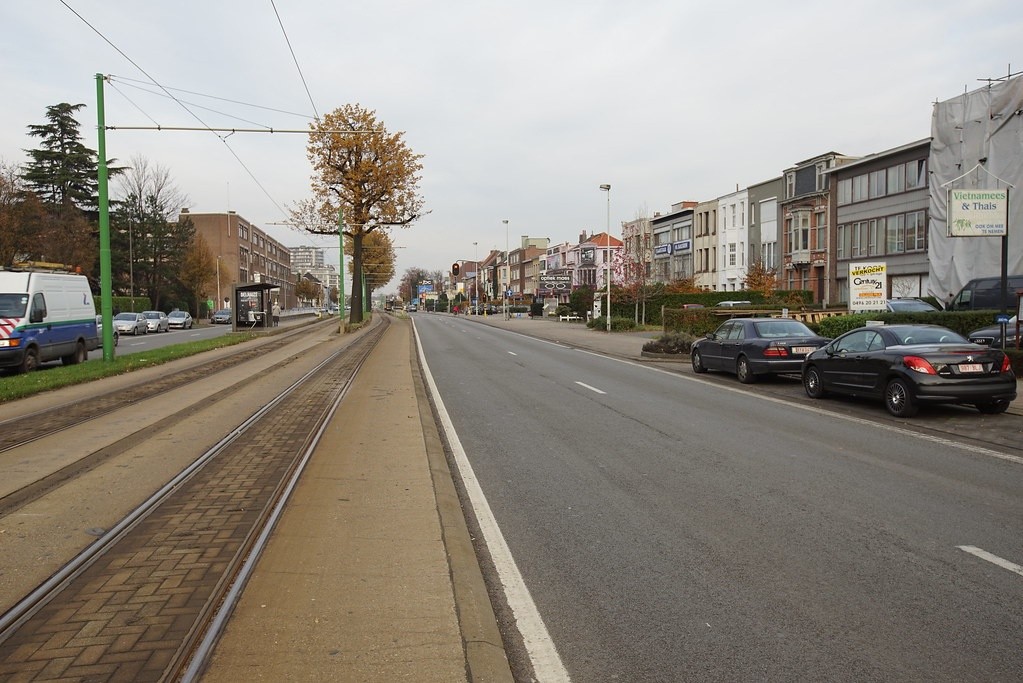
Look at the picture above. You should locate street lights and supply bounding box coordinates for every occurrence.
[502,217,510,322]
[127,201,135,312]
[472,240,479,317]
[216,255,222,311]
[283,267,287,309]
[598,182,611,331]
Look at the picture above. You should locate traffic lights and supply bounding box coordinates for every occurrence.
[482,292,487,305]
[452,263,460,277]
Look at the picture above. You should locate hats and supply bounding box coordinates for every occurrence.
[274,302,278,304]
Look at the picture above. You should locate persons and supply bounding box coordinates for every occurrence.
[453,304,458,316]
[271,302,281,327]
[466,305,471,315]
[5,297,25,318]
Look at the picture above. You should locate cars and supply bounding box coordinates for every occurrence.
[853,296,941,316]
[690,318,834,384]
[97,314,120,347]
[114,312,148,337]
[803,324,1018,417]
[142,310,169,334]
[211,310,232,324]
[714,300,752,307]
[168,311,193,329]
[967,314,1023,349]
[681,304,706,309]
[291,295,416,319]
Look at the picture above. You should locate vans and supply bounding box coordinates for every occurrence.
[944,274,1023,312]
[0,260,97,374]
